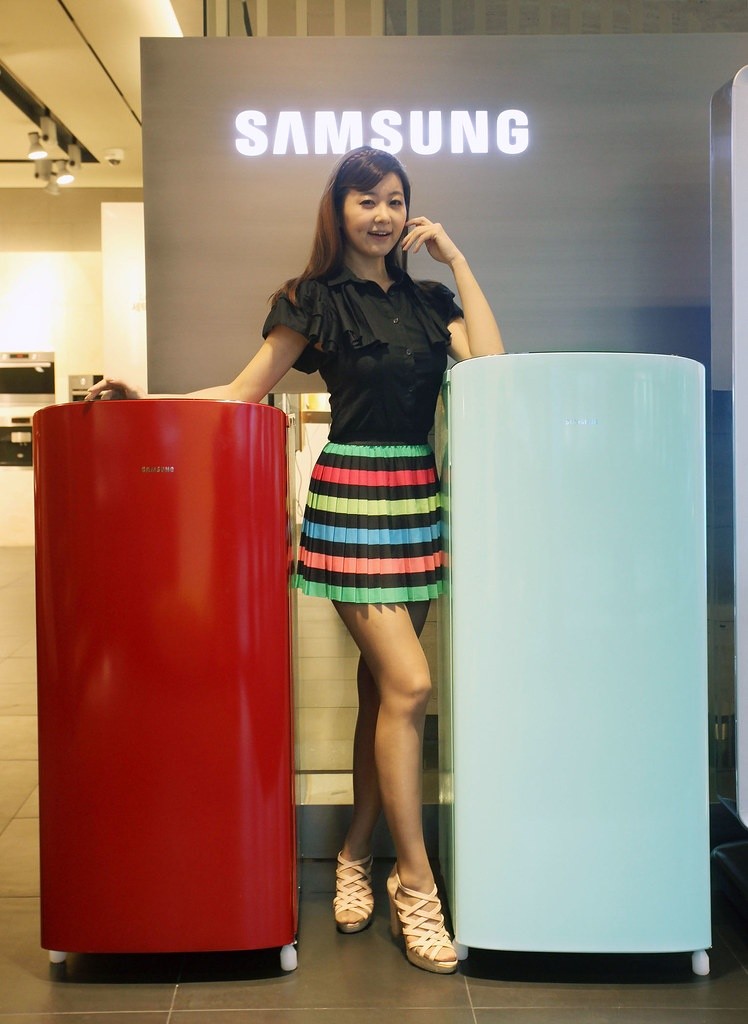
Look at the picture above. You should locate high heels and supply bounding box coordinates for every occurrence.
[333,851,374,933]
[387,862,458,973]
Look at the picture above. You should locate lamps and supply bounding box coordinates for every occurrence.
[28,117,58,160]
[57,145,82,186]
[33,160,61,196]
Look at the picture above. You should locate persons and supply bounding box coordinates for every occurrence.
[84,147,506,975]
